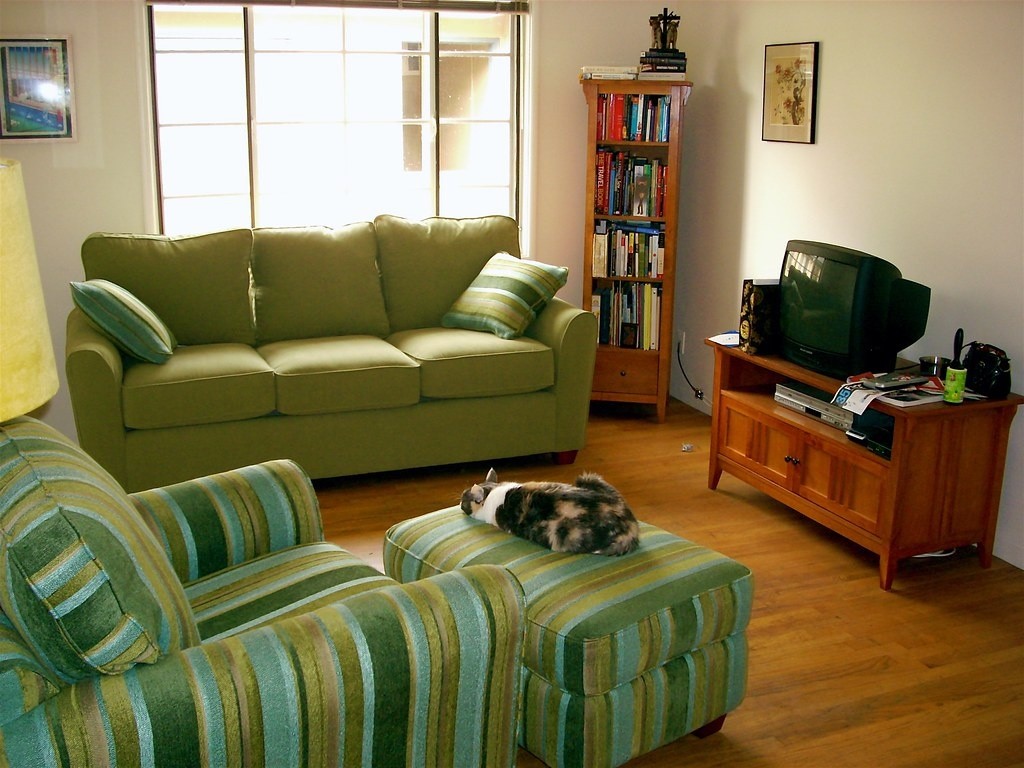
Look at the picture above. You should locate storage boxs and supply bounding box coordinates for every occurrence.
[739,278,780,356]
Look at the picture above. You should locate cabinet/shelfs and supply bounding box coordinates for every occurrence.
[579,79,695,422]
[703,338,1022,590]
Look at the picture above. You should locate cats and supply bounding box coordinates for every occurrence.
[458,467,641,557]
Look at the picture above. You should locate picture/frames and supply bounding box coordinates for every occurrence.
[762,42,819,144]
[0,32,78,143]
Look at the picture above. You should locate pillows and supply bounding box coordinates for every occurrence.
[442,250,570,340]
[68,280,178,366]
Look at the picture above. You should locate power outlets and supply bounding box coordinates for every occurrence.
[677,328,684,356]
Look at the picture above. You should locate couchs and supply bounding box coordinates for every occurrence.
[64,216,598,494]
[0,416,527,768]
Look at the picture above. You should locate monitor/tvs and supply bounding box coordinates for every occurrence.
[772,240,931,383]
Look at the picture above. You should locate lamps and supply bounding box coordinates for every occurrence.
[0,156,60,424]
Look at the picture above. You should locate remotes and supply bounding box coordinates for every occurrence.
[863,373,929,390]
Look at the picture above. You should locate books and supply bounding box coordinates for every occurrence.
[595,146,668,218]
[591,280,660,351]
[591,221,666,279]
[596,94,672,143]
[846,372,987,408]
[577,48,687,81]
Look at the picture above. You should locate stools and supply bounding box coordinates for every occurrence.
[383,505,753,768]
